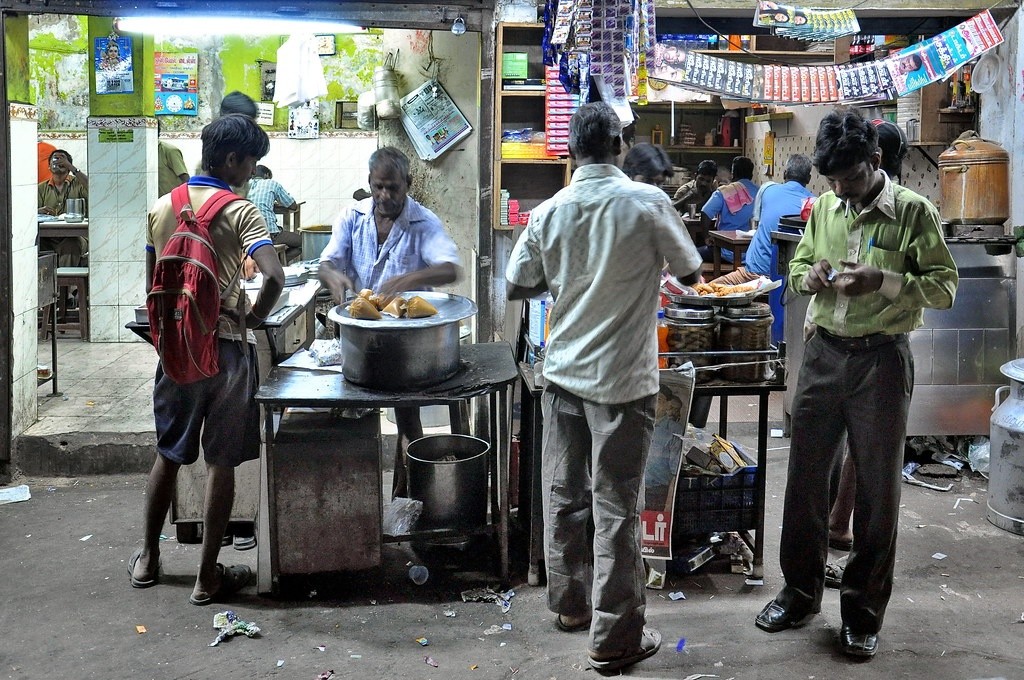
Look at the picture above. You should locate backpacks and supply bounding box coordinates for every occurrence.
[145,181,251,384]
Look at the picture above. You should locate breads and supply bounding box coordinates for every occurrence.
[693,282,754,297]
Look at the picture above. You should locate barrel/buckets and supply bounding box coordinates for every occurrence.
[986,359,1024,534]
[296,224,332,262]
[664,303,716,386]
[717,302,774,383]
[938,129,1010,226]
[405,433,491,533]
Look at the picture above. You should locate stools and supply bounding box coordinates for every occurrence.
[42,267,89,342]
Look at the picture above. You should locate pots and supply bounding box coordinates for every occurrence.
[327,291,478,394]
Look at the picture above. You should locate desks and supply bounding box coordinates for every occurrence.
[40,202,519,596]
[681,216,803,285]
[521,360,788,587]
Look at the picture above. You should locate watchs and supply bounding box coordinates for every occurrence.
[73,169,80,175]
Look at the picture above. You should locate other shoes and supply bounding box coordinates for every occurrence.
[828,538,856,551]
[824,562,843,588]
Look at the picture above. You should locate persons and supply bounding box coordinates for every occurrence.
[886,54,923,92]
[758,0,807,26]
[38,90,461,604]
[649,42,686,83]
[504,101,958,671]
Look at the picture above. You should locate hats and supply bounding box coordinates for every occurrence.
[871,119,908,159]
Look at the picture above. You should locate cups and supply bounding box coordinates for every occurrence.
[705,135,713,147]
[688,204,696,219]
[66,197,86,223]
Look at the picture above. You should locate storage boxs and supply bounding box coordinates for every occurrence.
[502,142,559,160]
[673,441,760,542]
[503,52,528,79]
[500,189,530,226]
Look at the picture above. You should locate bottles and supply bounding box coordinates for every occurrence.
[656,310,669,369]
[373,64,401,120]
[652,125,664,145]
[850,34,875,64]
[656,33,750,51]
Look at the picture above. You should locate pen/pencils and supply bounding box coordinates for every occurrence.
[868,236,875,253]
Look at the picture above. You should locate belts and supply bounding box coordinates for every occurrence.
[817,325,896,353]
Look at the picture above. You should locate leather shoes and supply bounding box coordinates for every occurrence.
[841,621,878,656]
[755,598,811,633]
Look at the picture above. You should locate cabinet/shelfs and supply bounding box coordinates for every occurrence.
[906,109,975,170]
[493,21,746,230]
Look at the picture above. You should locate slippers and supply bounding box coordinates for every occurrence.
[189,562,250,606]
[588,629,662,669]
[556,613,591,632]
[127,548,162,588]
